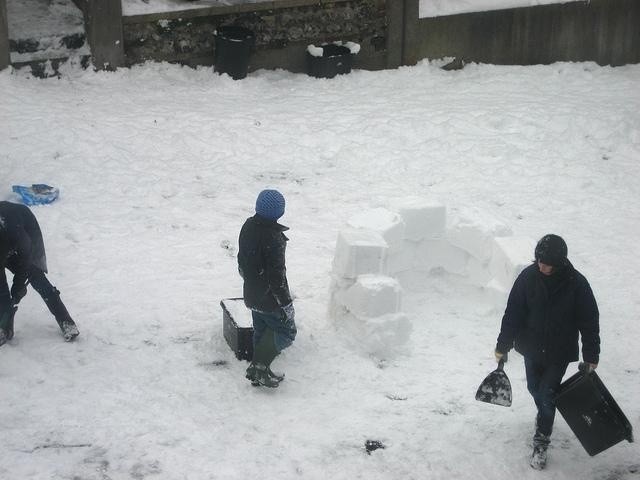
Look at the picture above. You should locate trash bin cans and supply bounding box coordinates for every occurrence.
[214,25,252,80]
[306,44,351,79]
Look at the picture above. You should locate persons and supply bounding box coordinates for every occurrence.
[0,201,79,347]
[494,234,600,470]
[237,189,297,389]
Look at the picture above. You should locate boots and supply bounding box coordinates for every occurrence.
[530,411,557,470]
[246,326,285,388]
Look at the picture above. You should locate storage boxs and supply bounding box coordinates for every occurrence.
[552,368,633,456]
[220,298,254,360]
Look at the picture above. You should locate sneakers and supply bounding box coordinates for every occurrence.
[57,315,82,342]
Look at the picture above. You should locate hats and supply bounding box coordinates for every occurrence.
[255,189,286,219]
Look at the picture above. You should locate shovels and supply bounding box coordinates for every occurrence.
[0,280,30,347]
[475,358,512,407]
